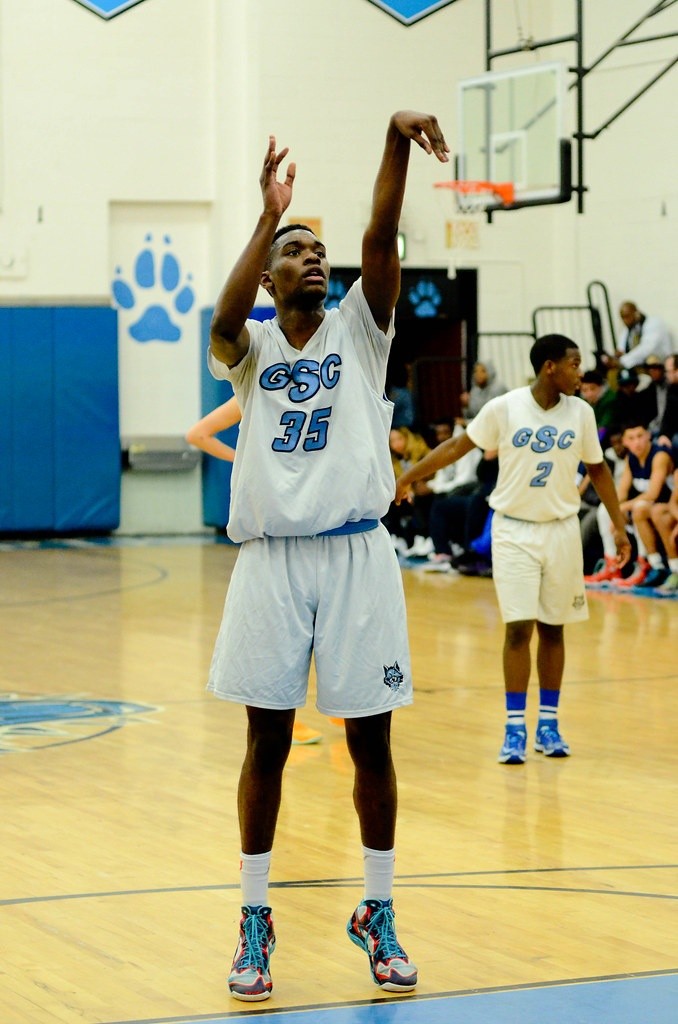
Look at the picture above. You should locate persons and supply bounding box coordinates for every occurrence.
[203,109,448,1002]
[186,301,678,597]
[398,333,628,766]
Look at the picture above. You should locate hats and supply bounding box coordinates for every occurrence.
[617,367,638,386]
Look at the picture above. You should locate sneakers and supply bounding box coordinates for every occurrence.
[610,557,652,589]
[500,723,527,764]
[583,555,636,585]
[228,906,275,1001]
[534,722,569,757]
[387,531,490,578]
[346,899,418,993]
[655,572,678,596]
[635,566,672,587]
